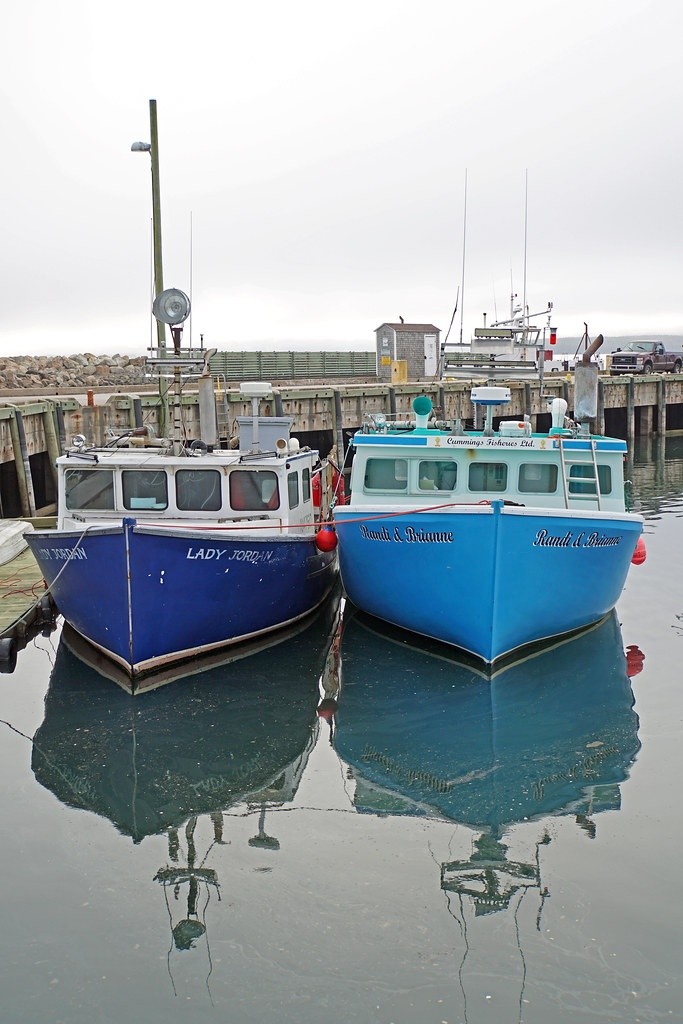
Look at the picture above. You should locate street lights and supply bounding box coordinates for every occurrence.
[132,142,167,393]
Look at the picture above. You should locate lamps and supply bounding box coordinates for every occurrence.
[72,435,86,454]
[153,288,191,358]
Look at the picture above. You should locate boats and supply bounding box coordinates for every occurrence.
[22,289,339,679]
[31,571,341,845]
[318,597,645,838]
[332,335,644,681]
[443,169,604,372]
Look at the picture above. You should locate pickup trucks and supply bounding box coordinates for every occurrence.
[610,340,683,376]
